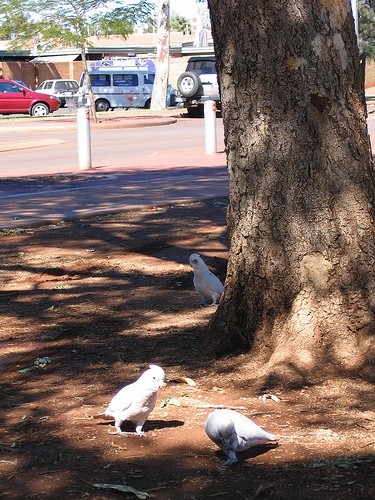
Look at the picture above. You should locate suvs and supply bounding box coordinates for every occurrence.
[173,54,223,117]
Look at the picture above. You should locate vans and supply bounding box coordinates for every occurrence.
[78,69,155,111]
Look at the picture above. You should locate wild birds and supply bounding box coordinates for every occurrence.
[204,408,280,466]
[190,253,224,306]
[92,364,163,438]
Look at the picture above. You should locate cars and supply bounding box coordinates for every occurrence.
[0,79,61,118]
[35,79,80,104]
